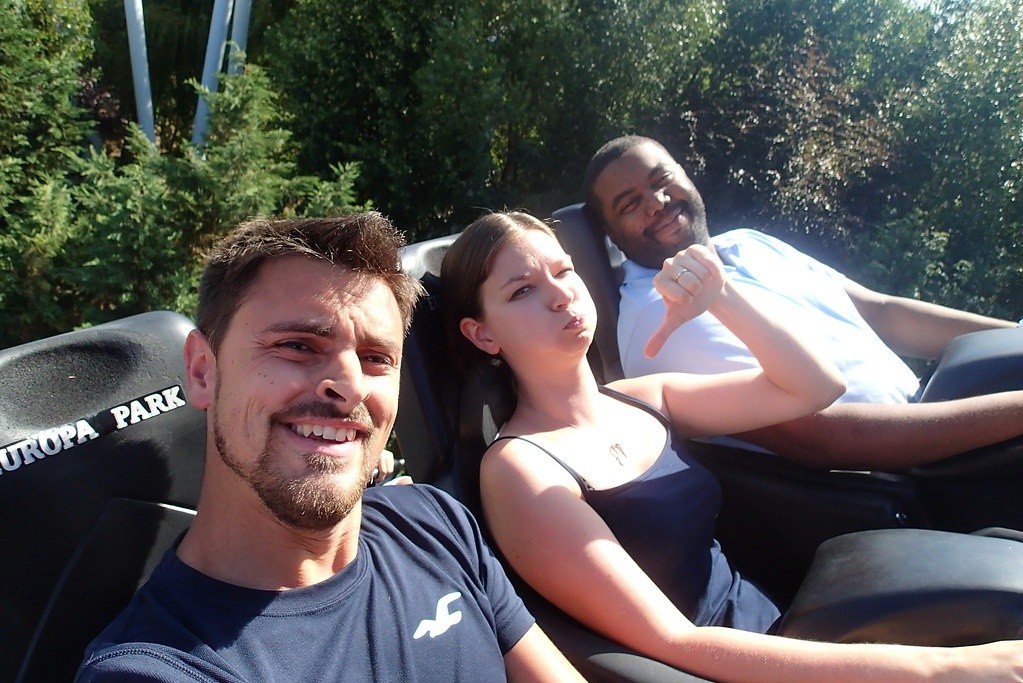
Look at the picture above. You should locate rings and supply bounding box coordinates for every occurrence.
[675,269,688,283]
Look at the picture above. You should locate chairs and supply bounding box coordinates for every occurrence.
[397,232,1023,648]
[0,311,711,683]
[551,202,1023,565]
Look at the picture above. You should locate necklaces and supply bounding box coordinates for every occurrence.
[521,399,631,458]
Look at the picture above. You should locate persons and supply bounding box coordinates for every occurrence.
[74,211,588,683]
[583,135,1023,468]
[419,211,1023,683]
